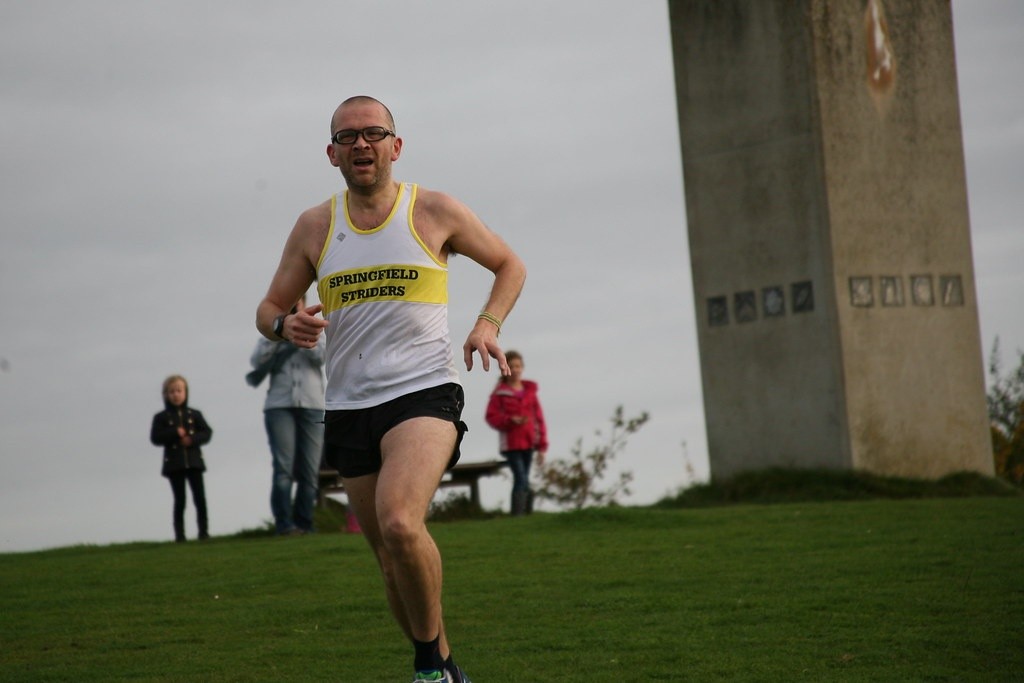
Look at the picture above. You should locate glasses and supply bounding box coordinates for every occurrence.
[332,125,396,145]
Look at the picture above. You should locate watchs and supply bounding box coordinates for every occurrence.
[270,314,288,339]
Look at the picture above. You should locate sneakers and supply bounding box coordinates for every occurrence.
[411,666,454,683]
[452,665,472,683]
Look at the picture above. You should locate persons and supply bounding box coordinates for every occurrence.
[485,351,548,516]
[249,291,326,526]
[257,96,526,683]
[151,374,213,542]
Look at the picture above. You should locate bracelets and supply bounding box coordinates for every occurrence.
[478,312,502,332]
